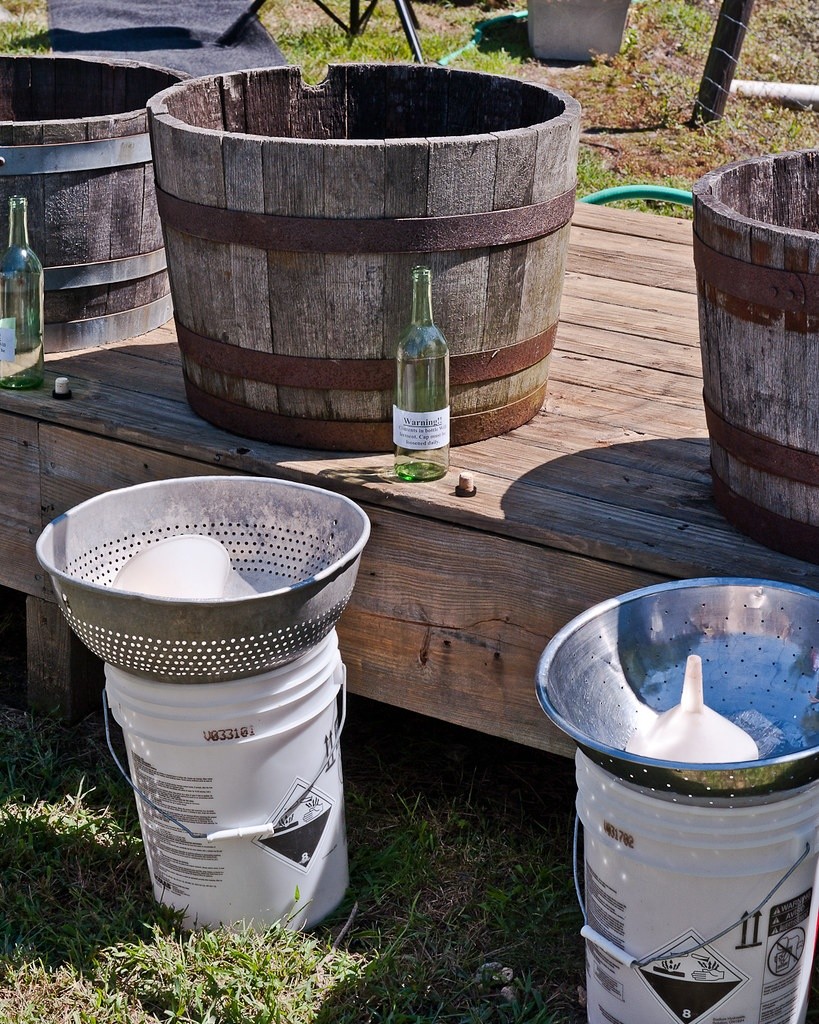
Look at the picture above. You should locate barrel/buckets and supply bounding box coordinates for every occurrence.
[573,747,819,1024]
[105,627,349,938]
[0,53,196,353]
[150,63,581,454]
[693,148,819,562]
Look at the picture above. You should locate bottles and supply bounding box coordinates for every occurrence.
[394,266,451,483]
[0,195,45,389]
